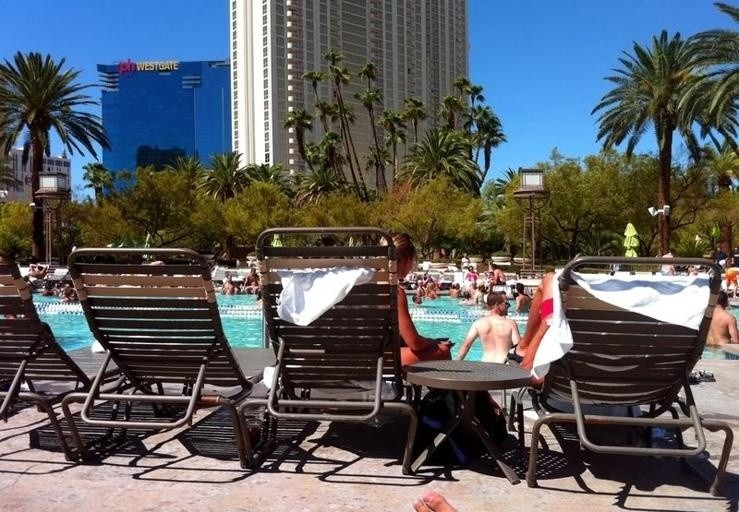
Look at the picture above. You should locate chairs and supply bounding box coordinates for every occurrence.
[18,263,48,292]
[504,254,732,496]
[0,250,122,460]
[69,248,277,468]
[238,226,417,474]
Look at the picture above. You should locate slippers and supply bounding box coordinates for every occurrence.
[687,370,716,384]
[507,347,524,362]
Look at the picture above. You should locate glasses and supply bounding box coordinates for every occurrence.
[499,300,509,305]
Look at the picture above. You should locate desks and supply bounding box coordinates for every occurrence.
[405,359,532,485]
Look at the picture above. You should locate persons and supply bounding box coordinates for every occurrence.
[401,252,532,314]
[23,261,167,303]
[384,231,502,420]
[507,272,556,390]
[413,490,457,512]
[658,246,739,347]
[221,249,262,303]
[457,290,522,366]
